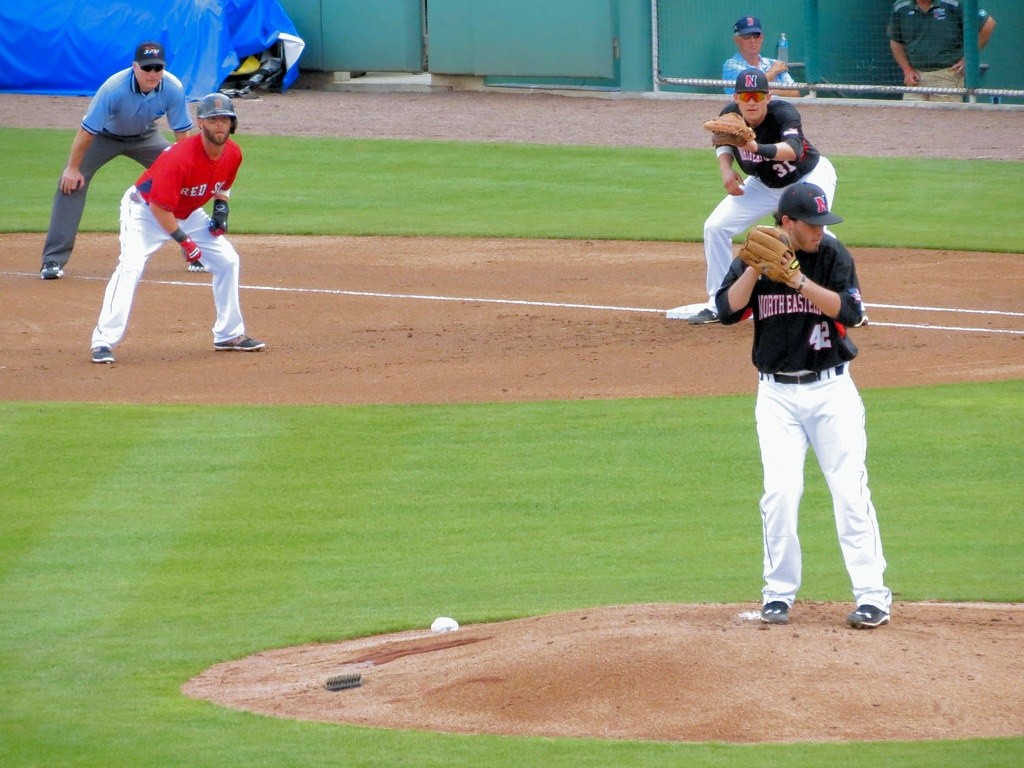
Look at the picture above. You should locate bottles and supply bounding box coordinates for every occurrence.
[778,32,789,63]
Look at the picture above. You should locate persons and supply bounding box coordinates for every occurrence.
[39,42,195,280]
[715,183,892,627]
[887,0,995,103]
[722,17,800,98]
[90,93,266,362]
[688,67,868,327]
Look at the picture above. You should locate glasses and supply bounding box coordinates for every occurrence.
[140,65,163,73]
[743,33,759,39]
[739,91,766,103]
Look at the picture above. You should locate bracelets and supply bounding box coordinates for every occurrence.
[753,144,777,159]
[798,274,807,291]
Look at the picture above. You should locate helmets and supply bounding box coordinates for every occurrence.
[197,93,238,135]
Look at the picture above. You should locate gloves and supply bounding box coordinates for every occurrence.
[209,199,229,236]
[179,235,201,263]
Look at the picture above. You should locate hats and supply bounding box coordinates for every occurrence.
[778,181,844,227]
[732,17,762,36]
[735,68,769,93]
[135,40,166,66]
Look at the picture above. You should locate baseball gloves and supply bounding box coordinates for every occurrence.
[737,225,801,282]
[703,112,757,149]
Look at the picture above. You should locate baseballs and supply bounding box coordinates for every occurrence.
[431,617,460,635]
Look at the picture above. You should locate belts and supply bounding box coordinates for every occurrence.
[761,364,843,385]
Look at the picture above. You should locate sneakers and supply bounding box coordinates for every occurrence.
[186,260,204,271]
[689,308,720,324]
[214,335,265,351]
[760,602,790,623]
[39,261,64,279]
[92,346,115,363]
[845,605,890,627]
